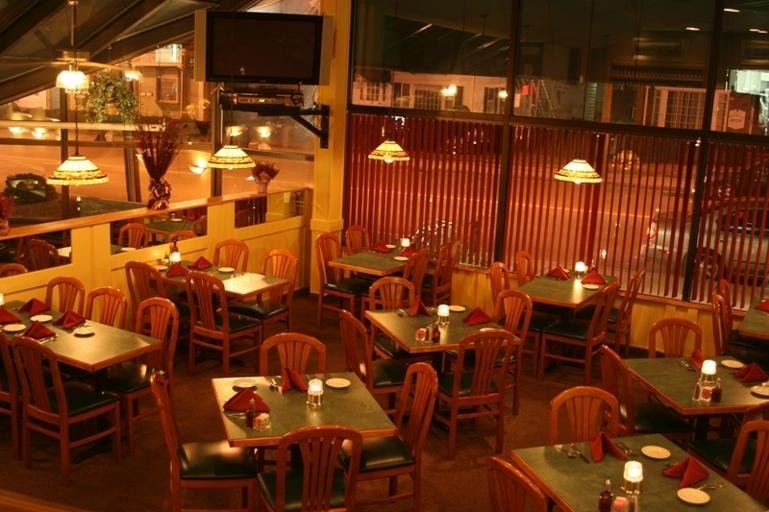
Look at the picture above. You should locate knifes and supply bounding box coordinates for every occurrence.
[570,443,590,465]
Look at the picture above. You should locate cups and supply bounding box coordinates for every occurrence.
[436,303,451,326]
[621,461,644,494]
[306,379,325,407]
[699,360,718,382]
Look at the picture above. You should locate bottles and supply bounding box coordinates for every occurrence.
[425,326,430,342]
[710,377,723,403]
[596,478,615,512]
[613,495,639,512]
[246,398,255,427]
[430,325,440,343]
[692,379,701,402]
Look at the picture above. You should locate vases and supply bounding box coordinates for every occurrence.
[147,178,173,211]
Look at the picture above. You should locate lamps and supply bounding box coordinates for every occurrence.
[360,2,411,165]
[51,63,88,100]
[52,3,109,187]
[204,6,260,172]
[552,3,605,187]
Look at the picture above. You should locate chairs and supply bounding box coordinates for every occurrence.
[364,251,769,512]
[1,225,462,511]
[0,193,249,278]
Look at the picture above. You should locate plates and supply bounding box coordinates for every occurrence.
[677,488,711,504]
[325,377,352,389]
[3,314,96,336]
[750,384,769,398]
[155,264,234,275]
[720,359,743,369]
[384,244,600,314]
[640,445,671,460]
[232,378,258,389]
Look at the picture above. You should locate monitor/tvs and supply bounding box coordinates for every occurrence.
[194,9,334,95]
